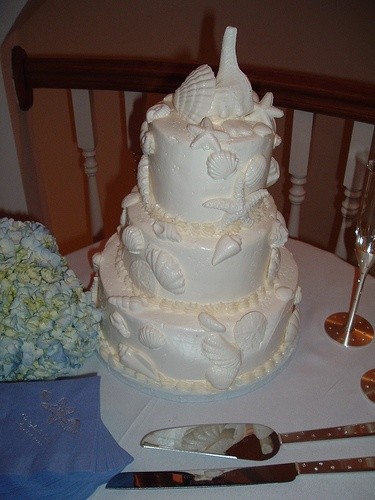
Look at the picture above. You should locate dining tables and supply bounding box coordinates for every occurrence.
[4,230,374,499]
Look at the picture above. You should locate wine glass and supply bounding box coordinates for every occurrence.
[324,160,375,346]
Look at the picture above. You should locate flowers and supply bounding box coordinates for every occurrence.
[0,217,104,382]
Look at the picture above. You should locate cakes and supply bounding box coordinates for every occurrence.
[89,25,307,402]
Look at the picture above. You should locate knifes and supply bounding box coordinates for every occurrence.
[105,457,375,490]
